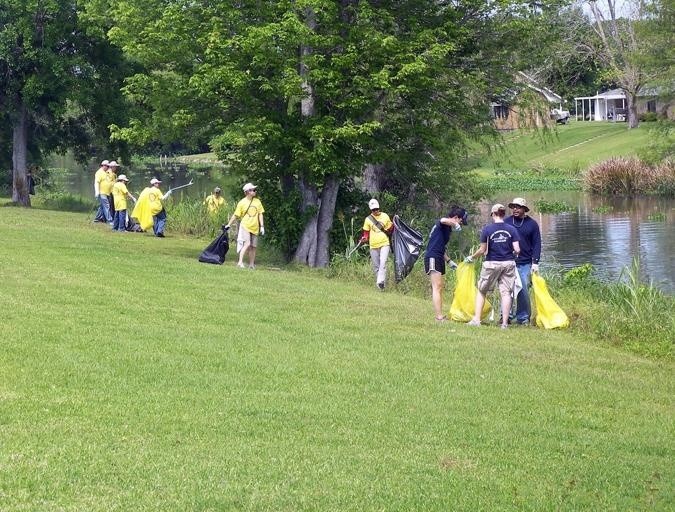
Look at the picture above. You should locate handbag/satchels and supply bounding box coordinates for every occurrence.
[387,232,395,254]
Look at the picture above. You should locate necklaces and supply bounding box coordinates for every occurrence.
[512,216,525,228]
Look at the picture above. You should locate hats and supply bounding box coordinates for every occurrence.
[150,178,162,185]
[117,174,129,182]
[100,159,120,167]
[490,203,506,217]
[242,182,258,192]
[214,187,221,193]
[368,198,380,211]
[508,197,530,213]
[449,208,469,226]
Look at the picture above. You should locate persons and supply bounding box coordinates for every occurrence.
[608,107,614,119]
[465,203,520,330]
[202,187,227,214]
[222,182,265,271]
[92,157,167,237]
[423,206,469,321]
[360,199,394,289]
[500,197,542,326]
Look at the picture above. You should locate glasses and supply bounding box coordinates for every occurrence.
[372,208,379,212]
[249,189,256,192]
[510,204,525,210]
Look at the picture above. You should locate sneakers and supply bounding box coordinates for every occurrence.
[236,262,257,270]
[465,318,482,327]
[499,323,508,329]
[435,315,450,322]
[157,231,166,238]
[378,282,385,292]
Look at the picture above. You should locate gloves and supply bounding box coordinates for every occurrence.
[451,222,462,233]
[259,226,266,235]
[225,225,232,233]
[464,256,474,264]
[132,197,137,203]
[531,263,540,275]
[447,259,458,270]
[359,236,367,243]
